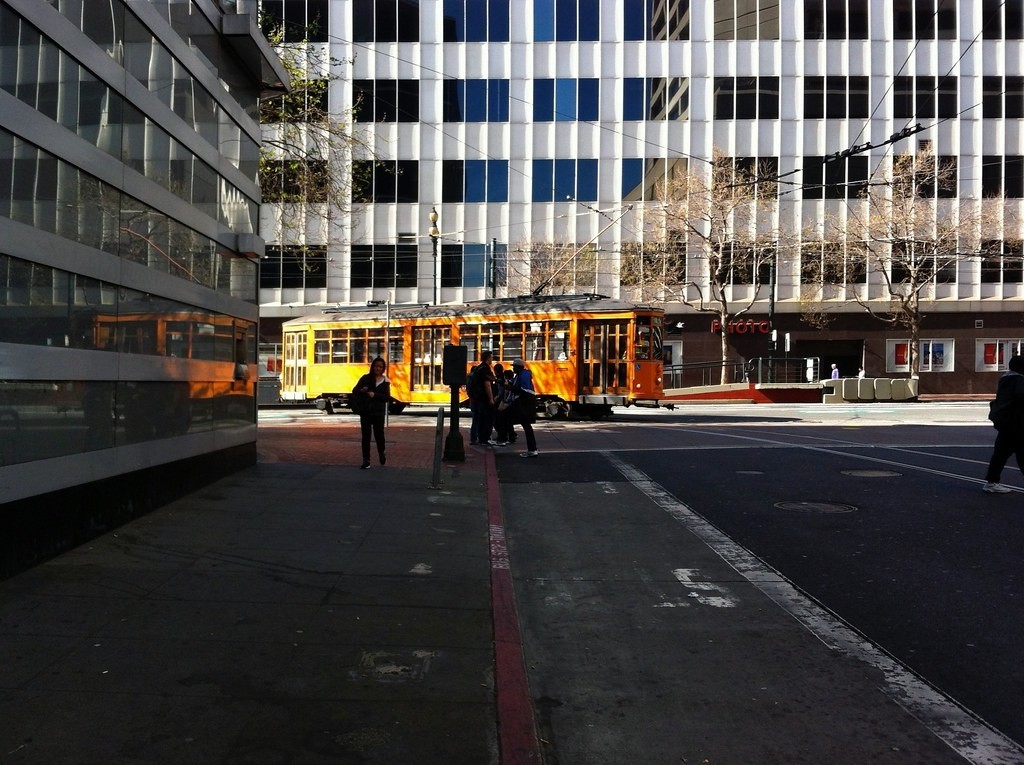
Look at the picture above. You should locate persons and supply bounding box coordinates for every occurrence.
[500,369,519,445]
[75,343,191,452]
[491,363,505,433]
[855,366,866,379]
[469,366,481,445]
[981,355,1024,494]
[352,357,390,470]
[831,364,839,379]
[473,350,496,449]
[505,358,539,457]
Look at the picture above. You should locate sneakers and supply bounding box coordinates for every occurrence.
[982,481,1013,492]
[508,431,519,442]
[379,454,387,465]
[520,449,539,458]
[360,461,370,469]
[492,435,507,445]
[483,440,498,446]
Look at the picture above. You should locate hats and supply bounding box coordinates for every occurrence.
[509,358,525,367]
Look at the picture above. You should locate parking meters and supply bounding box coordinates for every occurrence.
[442,345,468,462]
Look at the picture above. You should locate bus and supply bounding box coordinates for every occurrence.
[92,311,257,418]
[277,291,666,422]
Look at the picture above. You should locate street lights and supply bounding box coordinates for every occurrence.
[428,204,440,306]
